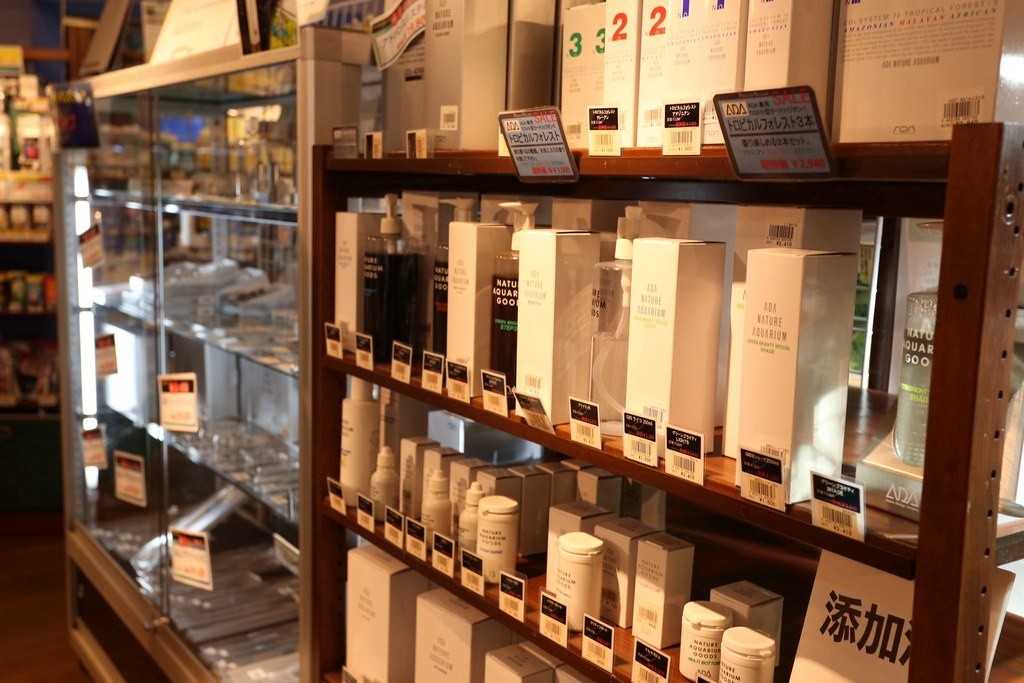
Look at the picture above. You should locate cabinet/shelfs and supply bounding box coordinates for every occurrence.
[1,172,63,510]
[50,25,371,683]
[313,122,1023,683]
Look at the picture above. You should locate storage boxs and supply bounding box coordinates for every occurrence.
[334,0,1023,683]
